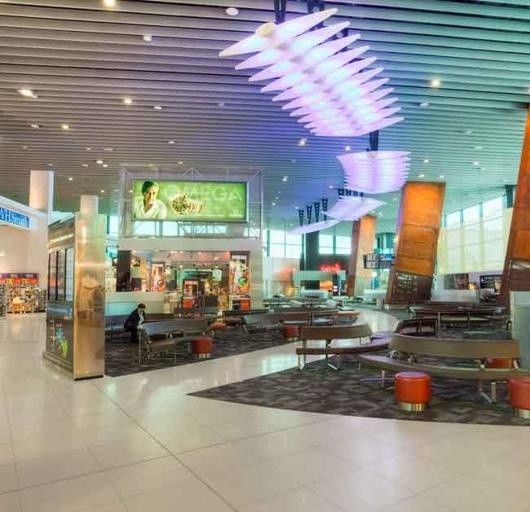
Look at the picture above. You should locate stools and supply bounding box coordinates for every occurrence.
[510,374,530,420]
[395,370,432,413]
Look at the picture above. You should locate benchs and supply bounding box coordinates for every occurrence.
[356,332,521,403]
[135,307,392,371]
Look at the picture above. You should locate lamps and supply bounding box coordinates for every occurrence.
[217,2,413,196]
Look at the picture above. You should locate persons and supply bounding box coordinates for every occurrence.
[124,304,147,343]
[133,181,167,219]
[115,271,130,291]
